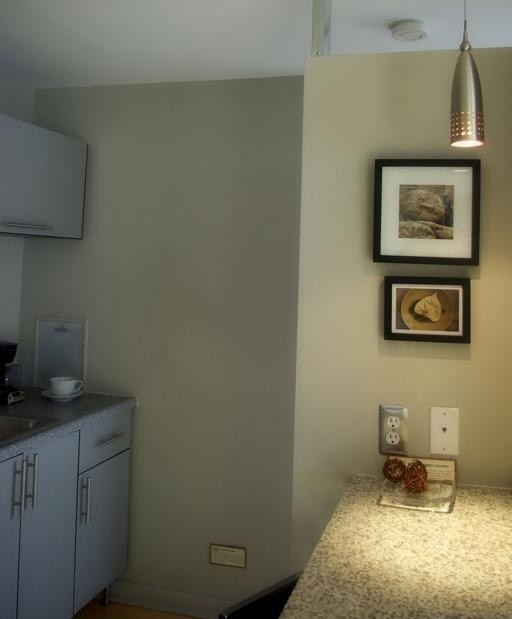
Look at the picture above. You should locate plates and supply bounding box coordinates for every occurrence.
[42,389,84,402]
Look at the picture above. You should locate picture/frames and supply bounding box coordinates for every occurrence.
[374,160,480,266]
[384,275,470,345]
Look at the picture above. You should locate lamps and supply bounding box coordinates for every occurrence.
[450,0,485,148]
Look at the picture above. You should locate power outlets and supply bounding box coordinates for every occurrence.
[378,404,408,457]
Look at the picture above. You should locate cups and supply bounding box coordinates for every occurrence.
[49,376,83,396]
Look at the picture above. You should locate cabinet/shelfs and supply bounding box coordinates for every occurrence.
[218,571,303,619]
[0,429,79,619]
[74,408,133,614]
[1,111,88,240]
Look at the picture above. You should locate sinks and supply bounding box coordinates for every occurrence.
[0,414,49,445]
